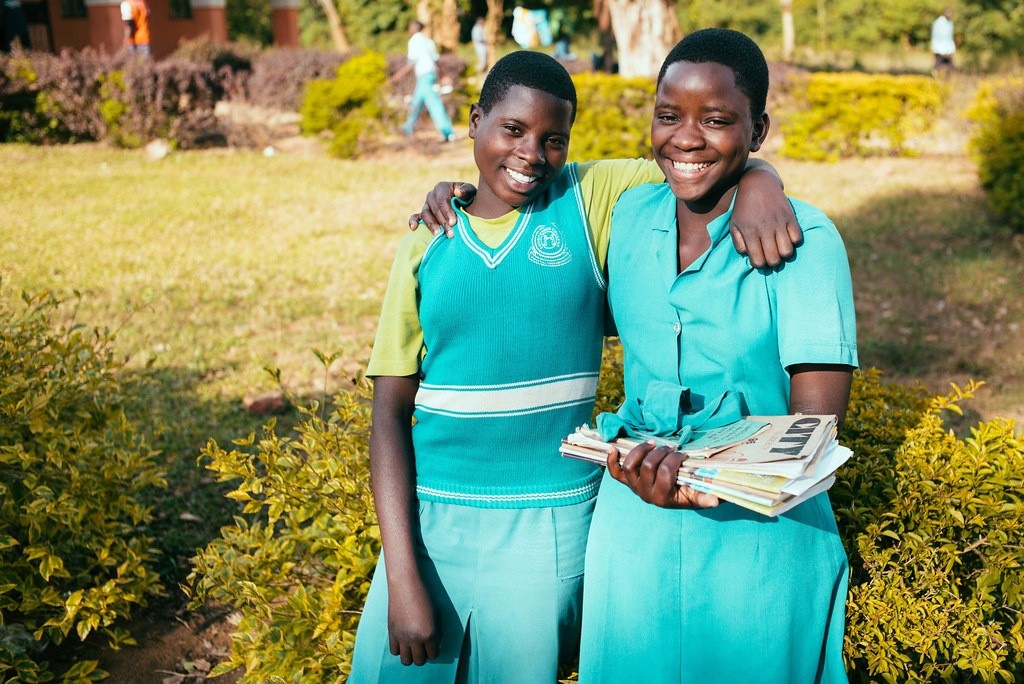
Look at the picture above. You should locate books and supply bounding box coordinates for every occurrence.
[558,413,853,517]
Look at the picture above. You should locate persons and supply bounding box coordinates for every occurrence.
[930,7,956,79]
[411,29,857,684]
[402,0,686,141]
[347,52,802,684]
[120,0,152,61]
